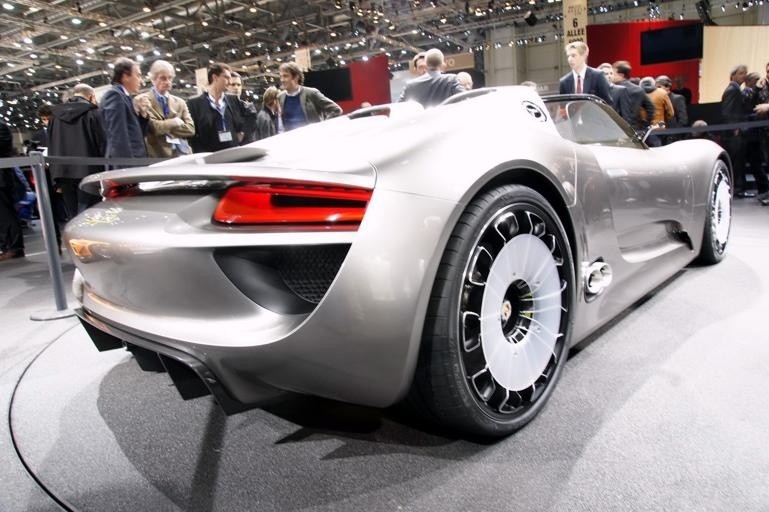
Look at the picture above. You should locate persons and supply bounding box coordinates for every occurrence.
[99,57,149,171]
[721,63,769,206]
[398,48,474,107]
[0,122,24,260]
[39,105,53,147]
[133,60,196,158]
[257,86,279,139]
[672,75,691,104]
[48,82,102,220]
[225,72,243,98]
[276,62,342,133]
[184,63,257,153]
[519,40,688,146]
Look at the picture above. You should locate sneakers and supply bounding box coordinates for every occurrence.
[0,249,25,260]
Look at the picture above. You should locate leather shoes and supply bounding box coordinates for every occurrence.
[758,196,768,206]
[735,192,756,198]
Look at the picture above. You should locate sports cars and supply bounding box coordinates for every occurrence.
[63,84,735,442]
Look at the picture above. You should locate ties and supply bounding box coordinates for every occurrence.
[162,97,166,104]
[577,75,581,93]
